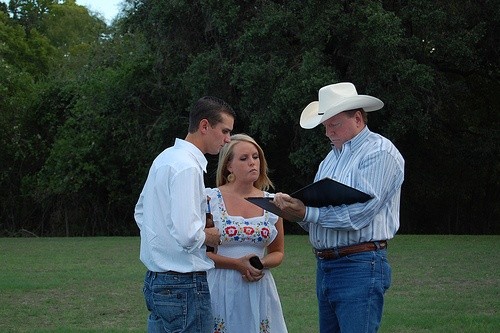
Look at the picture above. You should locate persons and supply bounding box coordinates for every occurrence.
[269,82,405,333]
[205,134,287,333]
[134,96,236,333]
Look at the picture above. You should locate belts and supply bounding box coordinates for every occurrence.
[315,240,388,260]
[167,270,206,275]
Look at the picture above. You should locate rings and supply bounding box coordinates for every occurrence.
[277,204,280,208]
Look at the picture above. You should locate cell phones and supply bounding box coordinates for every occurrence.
[249,256,263,270]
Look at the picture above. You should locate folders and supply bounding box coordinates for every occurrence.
[242,177,374,222]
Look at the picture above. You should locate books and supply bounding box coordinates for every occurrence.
[243,177,375,218]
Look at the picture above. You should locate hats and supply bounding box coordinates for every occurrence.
[299,82,385,130]
